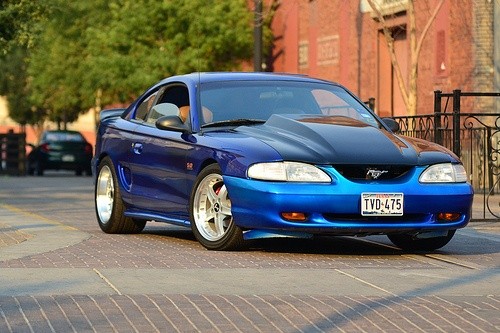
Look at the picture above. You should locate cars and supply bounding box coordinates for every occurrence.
[90,72,473,255]
[27,130,92,176]
